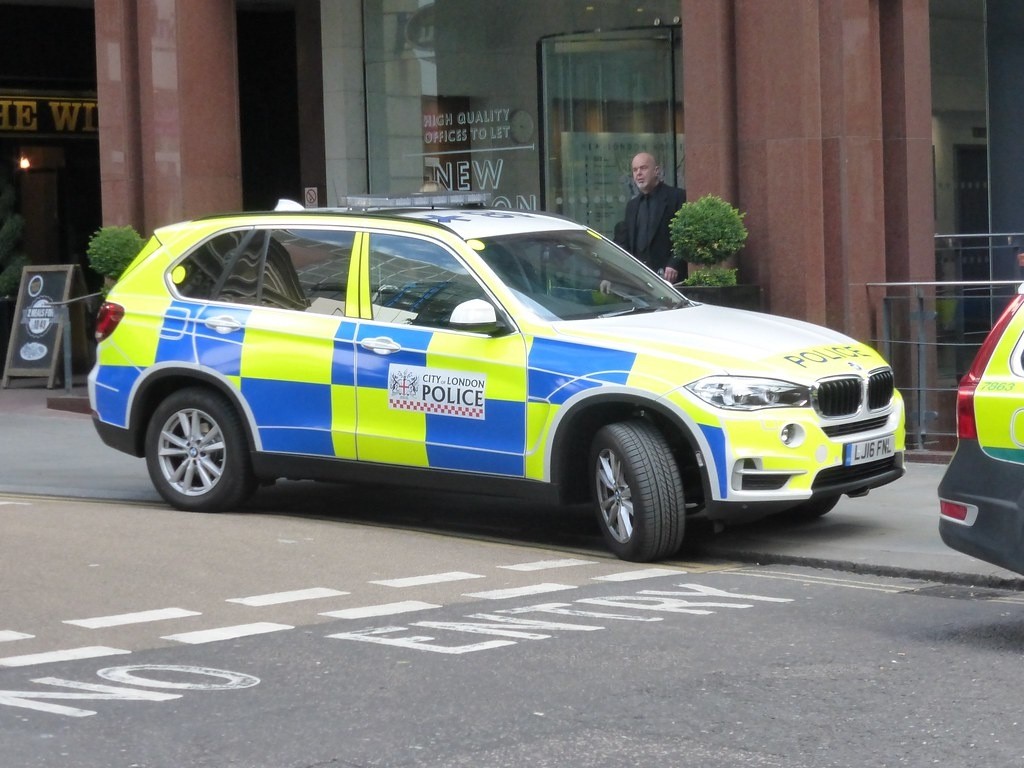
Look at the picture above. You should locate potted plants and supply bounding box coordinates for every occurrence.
[669,194,740,278]
[85,224,147,288]
[673,266,748,309]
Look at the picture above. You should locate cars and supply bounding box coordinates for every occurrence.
[934,281,1024,576]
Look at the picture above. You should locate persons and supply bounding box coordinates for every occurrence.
[600,152,688,294]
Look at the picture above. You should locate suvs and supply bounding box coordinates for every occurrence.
[86,197,908,564]
